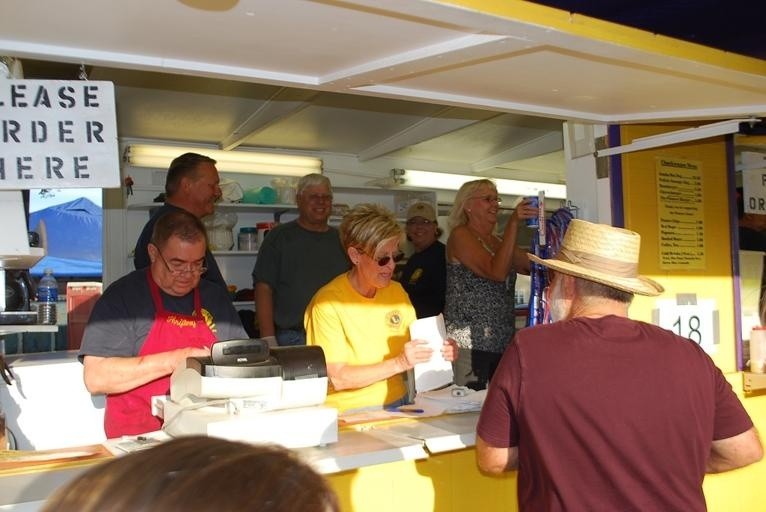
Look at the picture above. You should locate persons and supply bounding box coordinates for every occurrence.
[255,173,359,351]
[443,177,537,389]
[471,218,766,512]
[391,203,448,316]
[134,152,230,300]
[37,437,350,512]
[83,211,248,438]
[305,203,460,411]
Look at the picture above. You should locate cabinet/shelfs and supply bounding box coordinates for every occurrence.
[127,197,407,310]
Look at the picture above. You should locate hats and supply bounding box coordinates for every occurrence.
[407,202,436,221]
[527,219,665,296]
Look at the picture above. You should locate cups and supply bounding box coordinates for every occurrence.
[747,326,766,374]
[523,196,541,229]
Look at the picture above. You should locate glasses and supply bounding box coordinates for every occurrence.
[467,196,501,204]
[151,242,208,276]
[357,247,405,265]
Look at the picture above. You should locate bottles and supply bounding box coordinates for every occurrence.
[237,227,258,253]
[36,268,58,326]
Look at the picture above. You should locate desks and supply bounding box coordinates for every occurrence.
[0,324,58,359]
